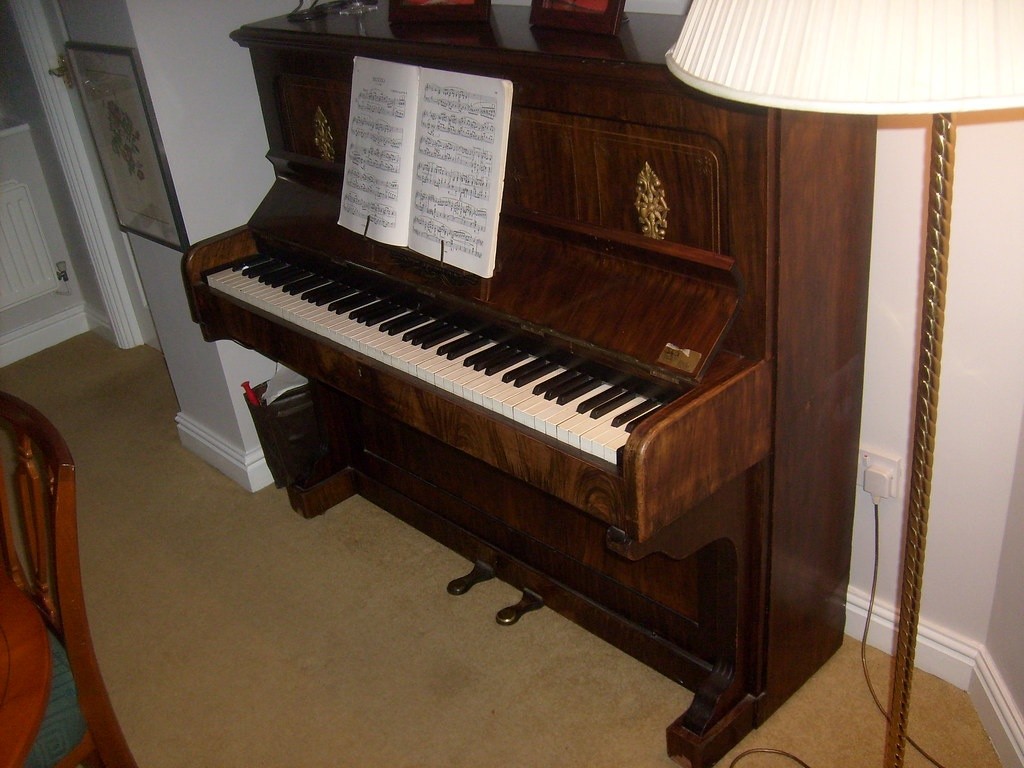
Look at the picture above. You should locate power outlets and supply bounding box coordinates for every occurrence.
[857,446,902,500]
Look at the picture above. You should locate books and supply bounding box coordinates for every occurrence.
[338,55,514,279]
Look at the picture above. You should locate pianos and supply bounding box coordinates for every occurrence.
[181,1,879,768]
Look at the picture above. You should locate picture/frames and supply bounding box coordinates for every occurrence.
[63,41,190,254]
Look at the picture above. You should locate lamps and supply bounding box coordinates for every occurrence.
[665,0,1024,768]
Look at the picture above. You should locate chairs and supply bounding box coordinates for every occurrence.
[0,392,138,768]
